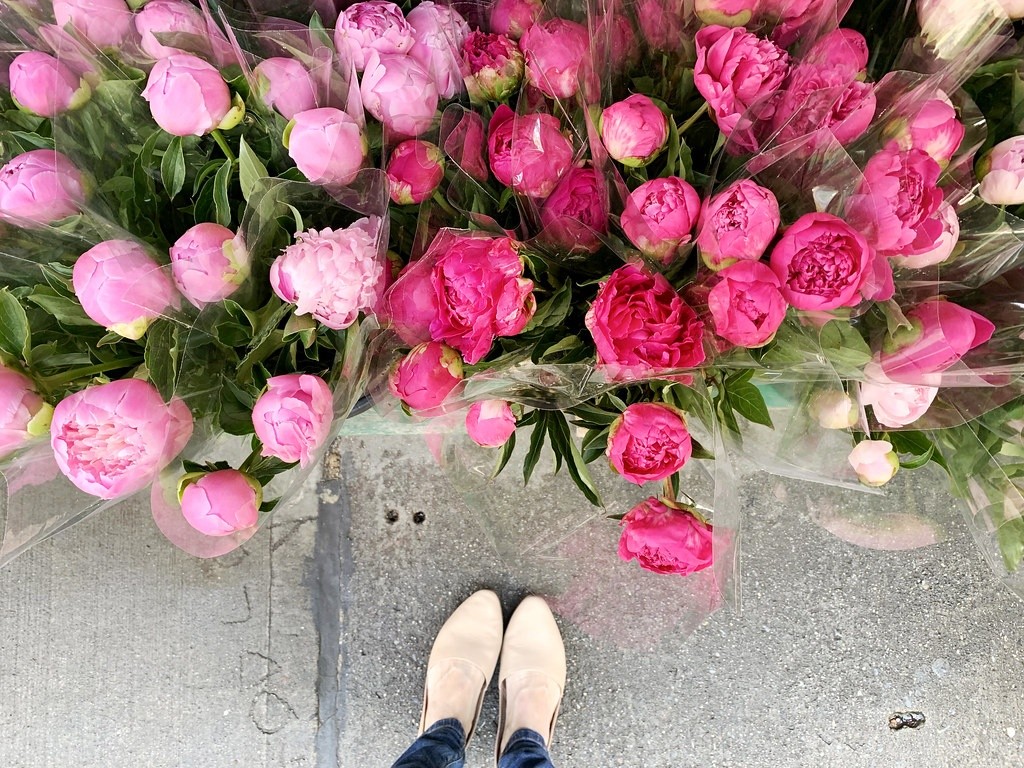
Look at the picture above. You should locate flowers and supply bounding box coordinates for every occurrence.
[0,0,1024,576]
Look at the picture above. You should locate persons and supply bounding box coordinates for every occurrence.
[391,590,566,768]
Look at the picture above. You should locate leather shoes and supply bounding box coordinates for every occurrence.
[494,594,567,768]
[418,589,504,753]
